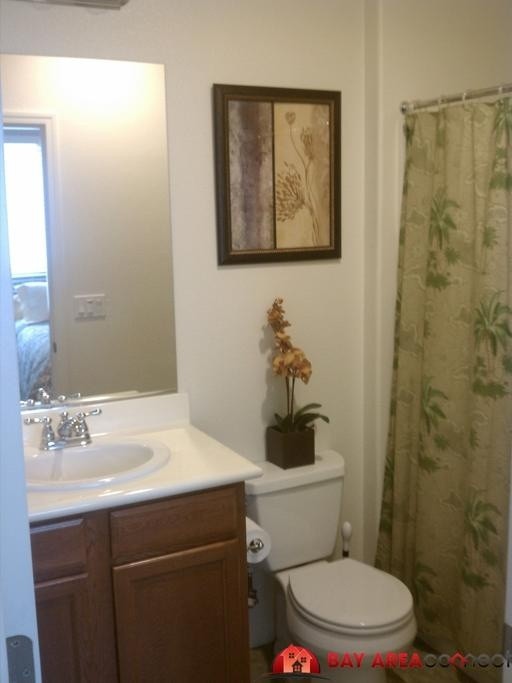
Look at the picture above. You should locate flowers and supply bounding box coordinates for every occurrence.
[264,293,330,432]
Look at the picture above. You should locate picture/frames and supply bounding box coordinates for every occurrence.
[210,79,344,268]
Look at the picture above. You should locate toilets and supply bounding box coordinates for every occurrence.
[244,449,419,683]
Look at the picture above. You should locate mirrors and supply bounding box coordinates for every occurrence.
[1,54,178,406]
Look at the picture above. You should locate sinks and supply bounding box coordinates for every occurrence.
[24,437,171,489]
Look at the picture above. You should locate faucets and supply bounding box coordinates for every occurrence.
[28,386,51,404]
[57,411,84,436]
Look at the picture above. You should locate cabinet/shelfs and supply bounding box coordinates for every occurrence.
[29,482,251,683]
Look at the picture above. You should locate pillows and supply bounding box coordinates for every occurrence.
[15,281,50,325]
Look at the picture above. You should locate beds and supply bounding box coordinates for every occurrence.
[12,275,51,404]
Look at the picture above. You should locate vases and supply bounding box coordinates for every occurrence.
[263,426,316,471]
